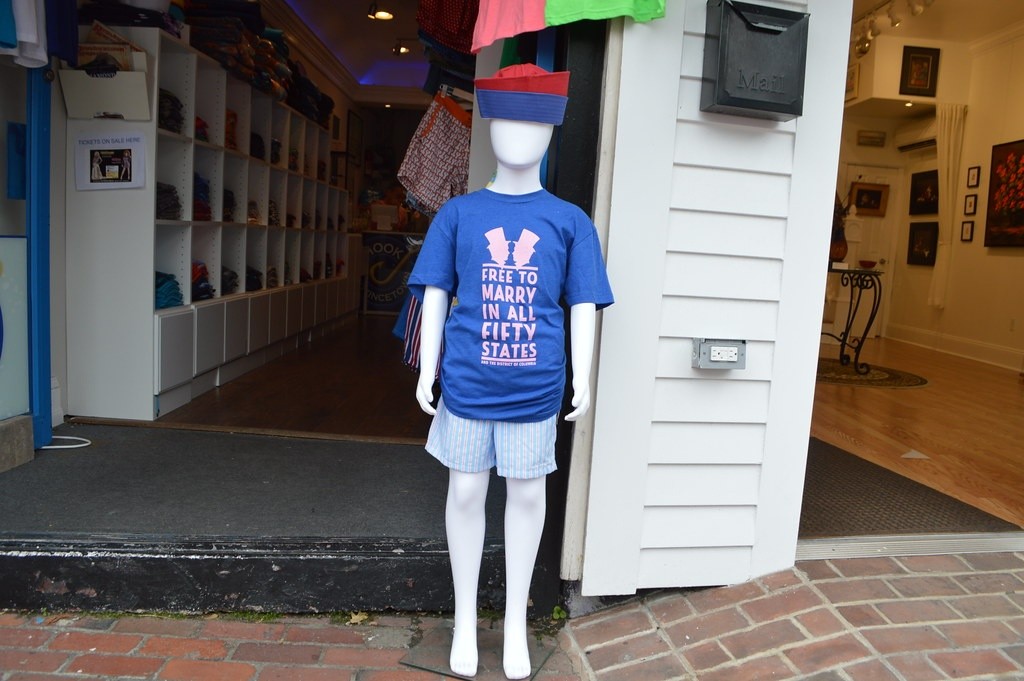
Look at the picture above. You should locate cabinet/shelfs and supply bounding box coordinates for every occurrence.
[67,24,363,421]
[821,268,885,375]
[439,83,473,110]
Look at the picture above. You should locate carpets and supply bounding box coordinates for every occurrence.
[799,437,1024,539]
[816,357,929,389]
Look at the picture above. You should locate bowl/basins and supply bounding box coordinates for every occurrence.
[859,260,877,269]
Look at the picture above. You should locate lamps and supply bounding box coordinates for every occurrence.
[368,2,375,20]
[393,39,402,55]
[852,0,934,58]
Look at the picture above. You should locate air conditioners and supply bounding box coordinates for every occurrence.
[895,116,937,156]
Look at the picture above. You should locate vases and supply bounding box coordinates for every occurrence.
[830,228,848,261]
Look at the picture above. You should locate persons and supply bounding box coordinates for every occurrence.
[406,61,615,680]
[92,150,131,180]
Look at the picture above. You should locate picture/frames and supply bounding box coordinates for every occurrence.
[961,221,973,241]
[848,182,890,217]
[845,63,858,102]
[967,166,979,187]
[907,221,938,266]
[964,194,976,215]
[984,139,1024,247]
[331,111,342,143]
[909,170,938,215]
[899,46,941,97]
